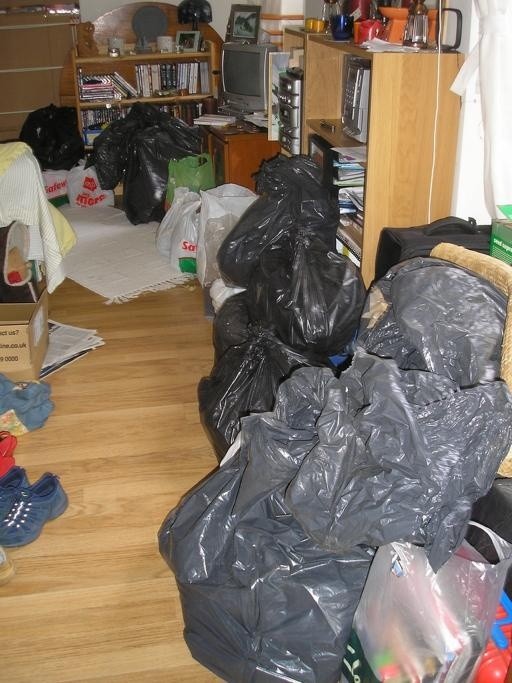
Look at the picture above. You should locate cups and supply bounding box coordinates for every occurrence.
[329,14,355,40]
[353,13,435,51]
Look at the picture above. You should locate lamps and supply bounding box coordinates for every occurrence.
[176,0,212,30]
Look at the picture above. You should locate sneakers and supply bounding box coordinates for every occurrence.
[0,470,70,548]
[0,464,29,522]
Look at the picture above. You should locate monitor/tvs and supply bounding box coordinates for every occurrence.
[217,42,279,113]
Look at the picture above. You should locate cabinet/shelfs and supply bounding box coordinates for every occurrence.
[1,15,75,115]
[280,27,465,291]
[64,41,220,151]
[205,122,279,196]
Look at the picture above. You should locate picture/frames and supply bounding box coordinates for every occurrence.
[230,5,261,43]
[176,30,201,51]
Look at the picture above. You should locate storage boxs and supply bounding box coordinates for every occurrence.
[1,278,50,385]
[375,218,493,283]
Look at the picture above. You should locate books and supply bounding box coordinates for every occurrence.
[193,112,236,125]
[77,60,210,126]
[332,152,365,232]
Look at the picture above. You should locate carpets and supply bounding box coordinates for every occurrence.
[57,199,201,306]
